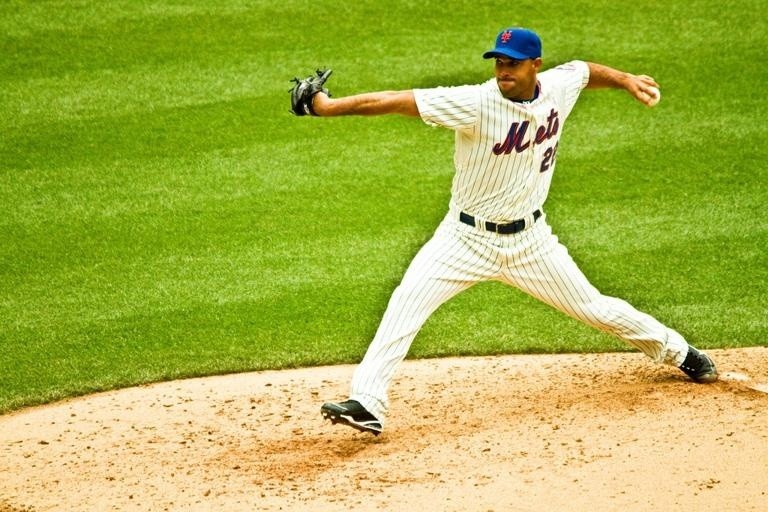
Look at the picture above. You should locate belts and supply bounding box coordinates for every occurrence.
[460,209,541,235]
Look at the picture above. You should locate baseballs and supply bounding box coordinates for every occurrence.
[642,86,660,107]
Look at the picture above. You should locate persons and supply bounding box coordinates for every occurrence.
[287,29,717,437]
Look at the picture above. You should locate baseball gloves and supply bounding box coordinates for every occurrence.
[291,70,332,115]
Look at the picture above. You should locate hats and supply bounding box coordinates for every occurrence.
[482,28,541,60]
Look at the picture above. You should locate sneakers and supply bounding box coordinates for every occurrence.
[321,400,382,436]
[679,346,718,382]
